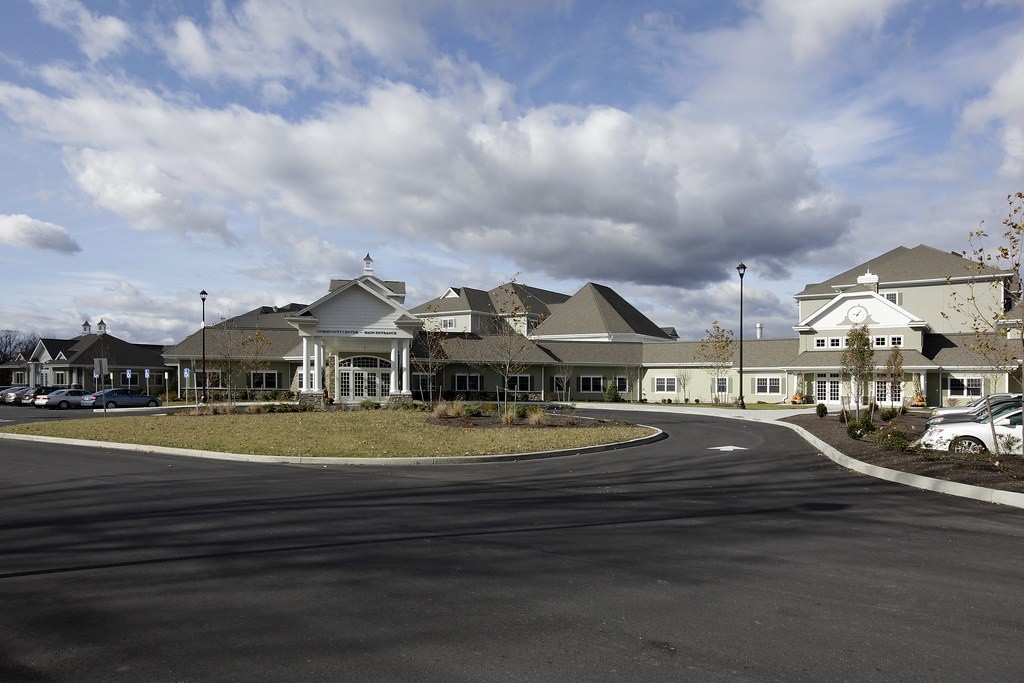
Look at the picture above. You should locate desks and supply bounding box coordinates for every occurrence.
[912,401,927,406]
[791,400,805,404]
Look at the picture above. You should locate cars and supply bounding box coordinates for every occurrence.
[21,386,67,408]
[923,406,1024,456]
[930,393,1024,418]
[0,385,17,404]
[34,388,93,410]
[925,396,1024,431]
[81,388,162,409]
[5,387,38,406]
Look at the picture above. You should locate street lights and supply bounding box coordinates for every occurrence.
[735,262,748,409]
[199,288,208,404]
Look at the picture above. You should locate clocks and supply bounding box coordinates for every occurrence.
[847,306,868,323]
